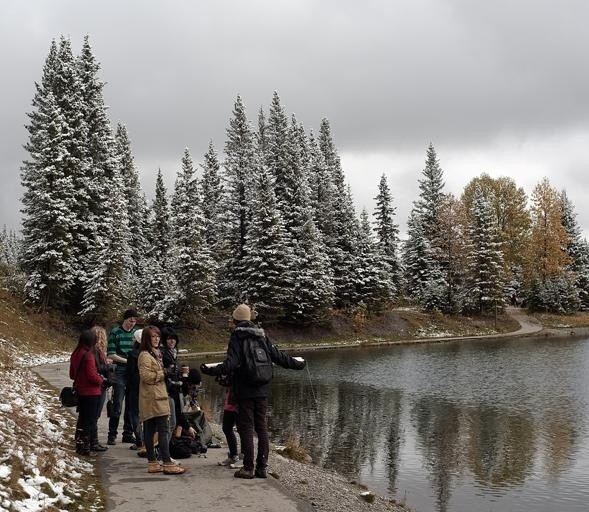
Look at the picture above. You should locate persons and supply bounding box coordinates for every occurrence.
[200,305,305,479]
[69,309,202,474]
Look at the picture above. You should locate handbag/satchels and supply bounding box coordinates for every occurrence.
[60,386,80,408]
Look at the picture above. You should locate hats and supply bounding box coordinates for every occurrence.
[123,309,139,320]
[232,303,252,322]
[134,329,145,344]
[186,368,202,383]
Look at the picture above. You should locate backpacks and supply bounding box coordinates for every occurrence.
[235,331,274,386]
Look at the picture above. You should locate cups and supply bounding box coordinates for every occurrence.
[182,365,189,378]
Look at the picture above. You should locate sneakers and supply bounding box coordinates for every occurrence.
[121,431,143,450]
[234,466,254,480]
[229,454,245,469]
[218,452,239,466]
[148,462,167,473]
[107,432,117,446]
[156,455,186,475]
[90,444,108,452]
[255,465,267,479]
[76,448,98,457]
